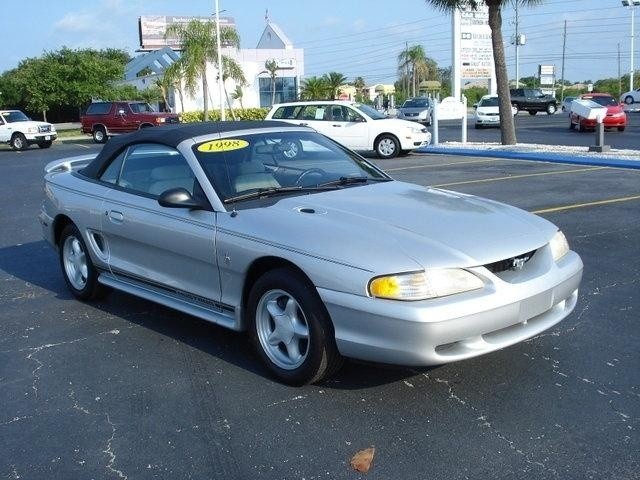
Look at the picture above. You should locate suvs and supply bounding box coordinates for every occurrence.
[0,110,57,151]
[81,101,180,143]
[510,88,557,115]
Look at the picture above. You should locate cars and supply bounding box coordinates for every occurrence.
[620,87,640,104]
[264,97,434,158]
[561,93,626,132]
[473,94,502,129]
[37,120,584,386]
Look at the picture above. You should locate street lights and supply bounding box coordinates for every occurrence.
[622,0,639,91]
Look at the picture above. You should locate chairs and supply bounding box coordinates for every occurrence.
[342,109,352,121]
[234,161,282,192]
[333,108,344,121]
[148,165,196,196]
